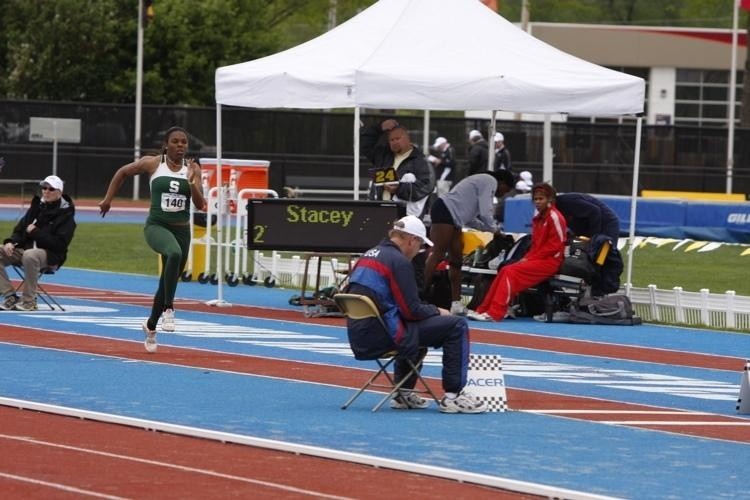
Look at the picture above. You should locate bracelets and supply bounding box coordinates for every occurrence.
[188,179,196,185]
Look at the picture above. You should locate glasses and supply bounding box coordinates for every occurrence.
[41,186,55,192]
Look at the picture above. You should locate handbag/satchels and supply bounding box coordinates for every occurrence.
[573,290,636,326]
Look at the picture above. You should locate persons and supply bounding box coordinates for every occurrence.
[97,126,207,352]
[492,132,513,172]
[342,215,489,414]
[0,175,77,312]
[427,137,458,184]
[555,192,620,297]
[467,181,566,322]
[360,119,436,227]
[421,169,518,316]
[467,130,488,174]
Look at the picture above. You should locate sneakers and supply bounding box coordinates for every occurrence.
[0,295,20,309]
[505,307,517,320]
[438,393,488,413]
[550,314,574,321]
[389,390,427,410]
[142,324,159,352]
[534,314,548,320]
[160,310,175,332]
[16,299,40,310]
[470,308,493,322]
[452,302,467,315]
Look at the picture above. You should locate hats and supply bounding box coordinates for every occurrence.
[495,133,504,142]
[391,216,435,247]
[433,138,447,148]
[520,171,534,186]
[40,175,64,192]
[469,130,481,139]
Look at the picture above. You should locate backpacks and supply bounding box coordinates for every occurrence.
[569,294,641,326]
[589,233,624,299]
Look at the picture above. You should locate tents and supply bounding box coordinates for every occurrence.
[214,0,644,309]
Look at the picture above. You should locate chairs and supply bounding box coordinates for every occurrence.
[334,292,441,413]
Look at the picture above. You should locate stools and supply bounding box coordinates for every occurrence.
[10,259,65,312]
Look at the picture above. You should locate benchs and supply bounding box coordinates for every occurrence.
[448,229,595,322]
